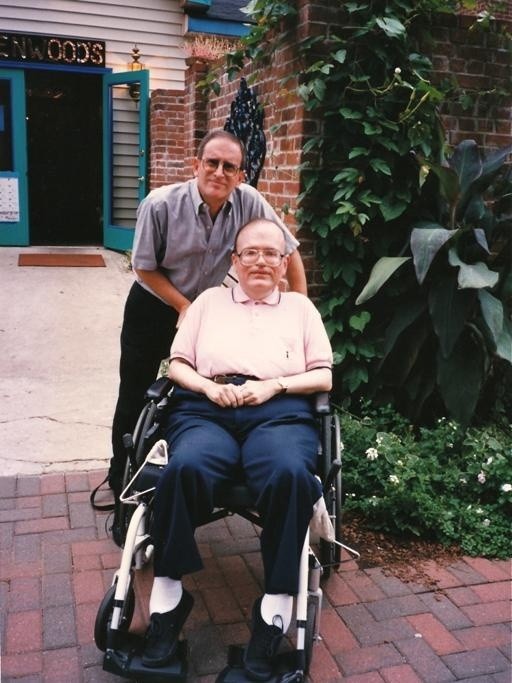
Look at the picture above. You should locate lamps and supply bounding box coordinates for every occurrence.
[127,42,146,109]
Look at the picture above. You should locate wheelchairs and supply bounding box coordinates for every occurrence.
[94,376,343,681]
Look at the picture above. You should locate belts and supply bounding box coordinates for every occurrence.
[209,373,263,386]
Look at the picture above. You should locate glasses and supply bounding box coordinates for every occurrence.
[232,247,286,267]
[198,156,243,177]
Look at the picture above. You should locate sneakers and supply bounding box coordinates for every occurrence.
[141,586,195,666]
[242,594,284,681]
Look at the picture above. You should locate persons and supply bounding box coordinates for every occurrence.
[140,220,335,679]
[104,129,309,544]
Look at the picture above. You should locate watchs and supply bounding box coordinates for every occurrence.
[277,376,289,393]
